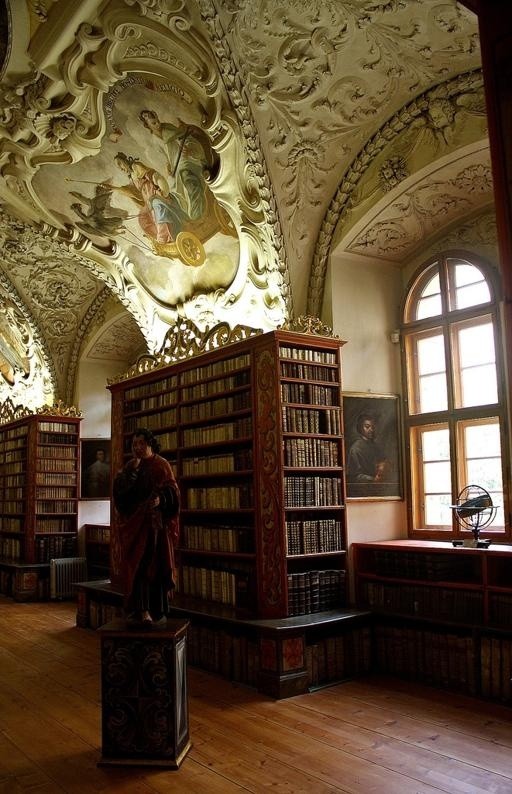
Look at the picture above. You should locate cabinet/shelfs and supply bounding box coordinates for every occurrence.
[69,314,373,702]
[1,395,80,600]
[352,536,512,704]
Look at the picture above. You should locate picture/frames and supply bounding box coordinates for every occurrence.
[77,437,113,501]
[339,390,410,503]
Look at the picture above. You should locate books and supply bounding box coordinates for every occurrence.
[0,422,77,597]
[123,355,250,473]
[357,550,512,705]
[181,487,246,606]
[278,345,354,685]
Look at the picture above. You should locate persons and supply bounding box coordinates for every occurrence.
[346,411,387,489]
[114,427,182,625]
[114,110,215,247]
